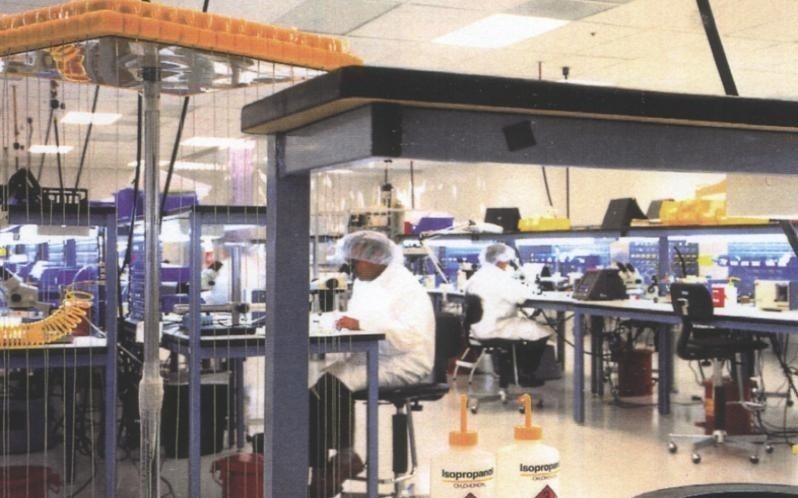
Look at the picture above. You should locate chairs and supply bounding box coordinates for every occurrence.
[348,311,459,494]
[669,283,775,465]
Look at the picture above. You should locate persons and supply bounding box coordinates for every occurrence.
[201,262,223,290]
[465,242,549,387]
[311,279,338,292]
[309,230,436,498]
[336,264,349,291]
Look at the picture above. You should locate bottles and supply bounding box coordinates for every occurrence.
[431,394,559,498]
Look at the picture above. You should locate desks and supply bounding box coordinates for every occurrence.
[424,285,797,423]
[119,299,386,497]
[1,318,119,497]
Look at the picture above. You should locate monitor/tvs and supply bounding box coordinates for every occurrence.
[573,270,609,299]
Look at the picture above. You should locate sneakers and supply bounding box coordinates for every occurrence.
[310,453,364,497]
[500,374,544,387]
